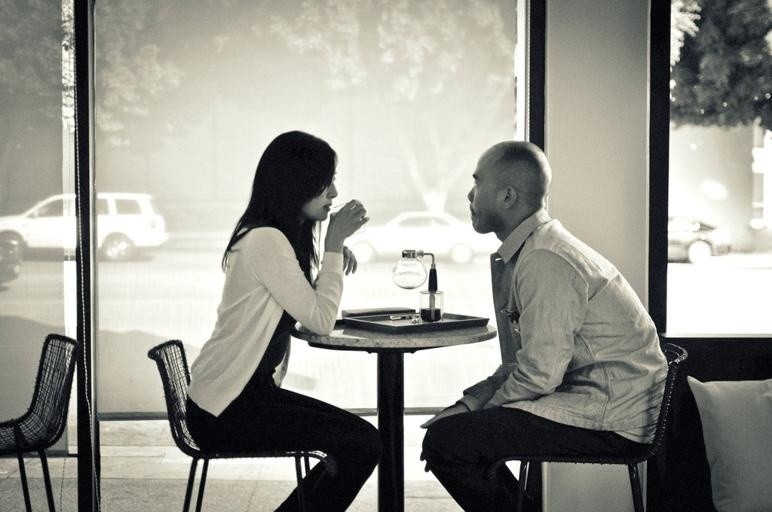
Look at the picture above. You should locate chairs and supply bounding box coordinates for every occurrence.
[145,337,336,507]
[484,341,693,506]
[2,331,81,506]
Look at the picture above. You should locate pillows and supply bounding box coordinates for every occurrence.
[682,373,771,507]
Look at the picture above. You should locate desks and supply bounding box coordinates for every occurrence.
[291,307,498,507]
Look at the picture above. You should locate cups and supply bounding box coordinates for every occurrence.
[330,200,365,233]
[419,289,444,324]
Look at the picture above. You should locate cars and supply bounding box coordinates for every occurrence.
[349,211,496,268]
[667,207,735,268]
[0,192,170,262]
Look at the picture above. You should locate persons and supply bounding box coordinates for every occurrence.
[421,142,669,511]
[186,131,379,511]
